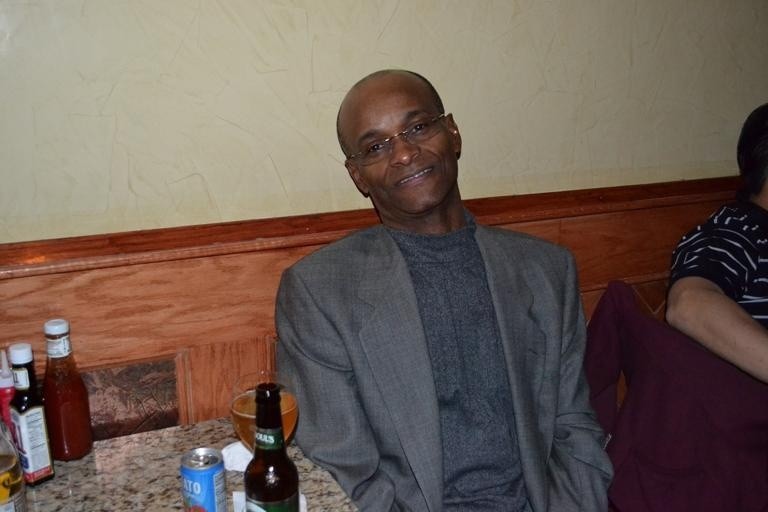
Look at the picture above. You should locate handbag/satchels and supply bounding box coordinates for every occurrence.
[587,279,768,512]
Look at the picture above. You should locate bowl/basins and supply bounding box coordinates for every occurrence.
[232,391,297,449]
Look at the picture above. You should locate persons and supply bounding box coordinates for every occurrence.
[275,68,615,512]
[662,102,767,384]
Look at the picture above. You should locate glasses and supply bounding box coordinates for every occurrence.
[346,114,445,160]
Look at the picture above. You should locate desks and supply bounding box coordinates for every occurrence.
[28,418,359,511]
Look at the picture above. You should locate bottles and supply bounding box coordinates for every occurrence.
[245,382,300,510]
[0,319,92,511]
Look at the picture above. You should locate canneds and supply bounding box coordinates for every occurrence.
[179,447,227,511]
